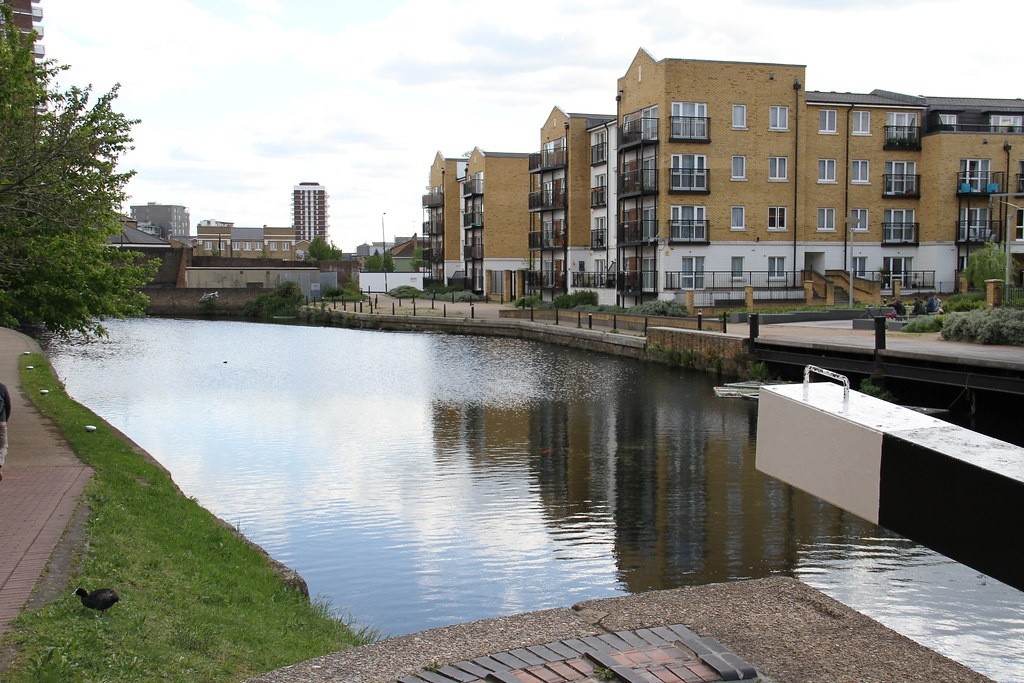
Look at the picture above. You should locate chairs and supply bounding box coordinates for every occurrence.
[960,183,973,193]
[987,183,999,193]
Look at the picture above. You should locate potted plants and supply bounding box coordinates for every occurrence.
[912,272,919,288]
[875,266,890,289]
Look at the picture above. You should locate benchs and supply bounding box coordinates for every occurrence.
[896,314,917,321]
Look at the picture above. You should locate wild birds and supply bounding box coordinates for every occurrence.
[71,587,119,617]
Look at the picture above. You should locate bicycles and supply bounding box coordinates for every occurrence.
[859,305,898,321]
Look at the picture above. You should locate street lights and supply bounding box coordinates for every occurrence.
[381,212,388,293]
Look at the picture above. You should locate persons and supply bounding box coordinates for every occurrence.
[924,295,941,315]
[909,297,925,315]
[0,383,11,481]
[886,298,906,316]
